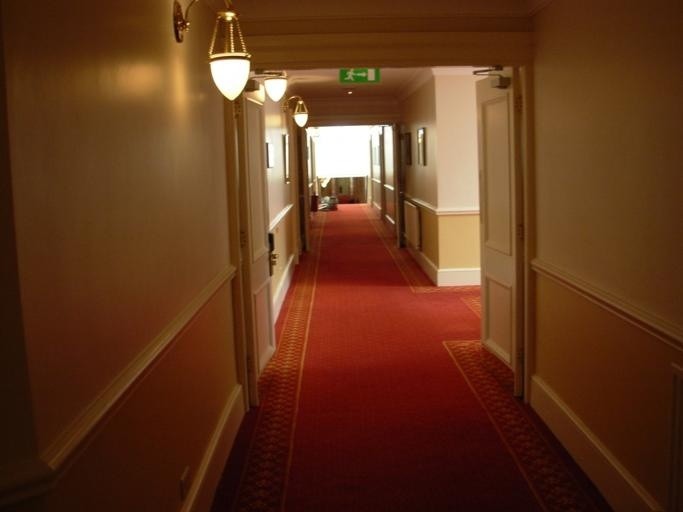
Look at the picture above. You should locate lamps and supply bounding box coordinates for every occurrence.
[171,1,308,129]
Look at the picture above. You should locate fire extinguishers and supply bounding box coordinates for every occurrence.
[310,191,319,212]
